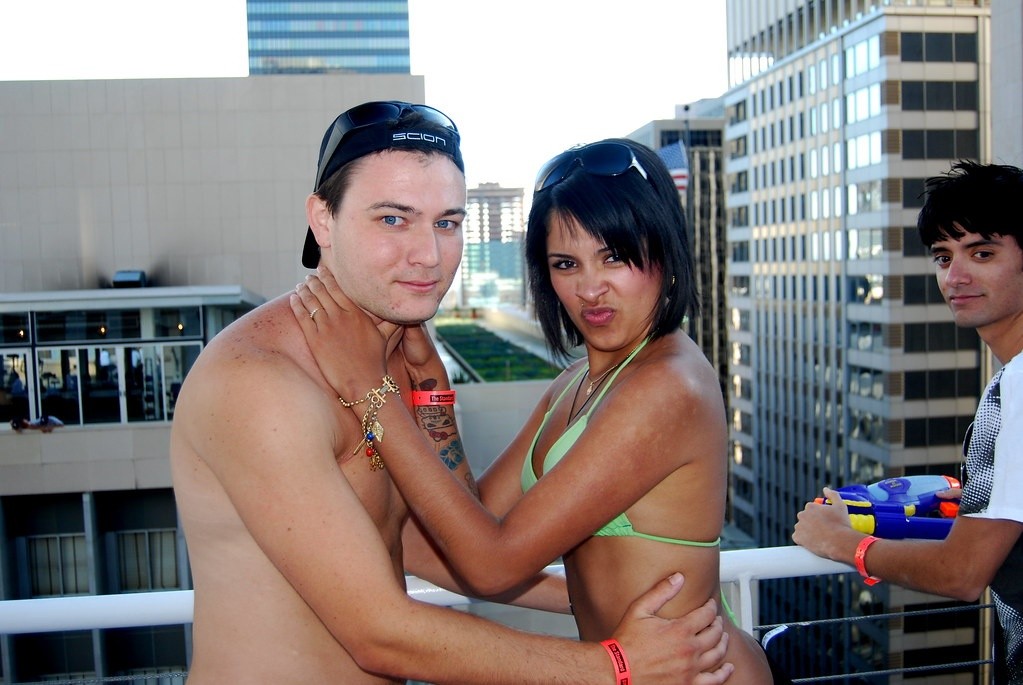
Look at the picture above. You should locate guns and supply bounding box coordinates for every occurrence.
[812,474,961,541]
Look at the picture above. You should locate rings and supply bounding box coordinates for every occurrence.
[310,307,323,318]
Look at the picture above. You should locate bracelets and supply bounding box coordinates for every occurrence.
[337,375,403,474]
[600,639,632,685]
[854,536,882,586]
[413,388,457,406]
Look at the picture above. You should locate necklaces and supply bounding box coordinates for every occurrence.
[586,341,641,396]
[566,354,629,426]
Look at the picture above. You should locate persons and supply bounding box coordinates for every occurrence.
[169,99,736,685]
[289,138,775,685]
[11,373,22,397]
[792,159,1022,684]
[10,415,64,433]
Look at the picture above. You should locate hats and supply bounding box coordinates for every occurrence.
[302,100,465,269]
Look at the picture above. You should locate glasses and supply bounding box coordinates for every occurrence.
[534,141,661,200]
[315,101,460,191]
[961,422,974,490]
